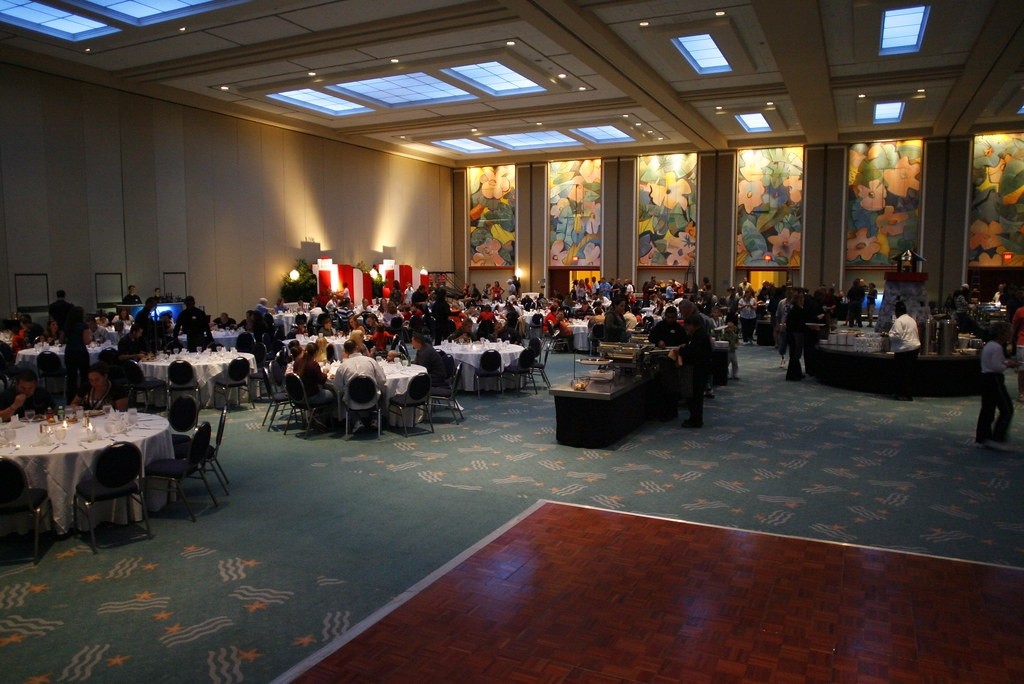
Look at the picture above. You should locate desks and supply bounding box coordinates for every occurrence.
[814,333,982,398]
[549,346,730,449]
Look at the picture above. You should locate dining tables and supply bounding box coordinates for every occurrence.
[0,410,175,535]
[14,344,118,395]
[177,331,256,351]
[281,336,348,363]
[566,321,592,350]
[272,313,310,337]
[136,352,261,409]
[433,343,525,391]
[320,361,428,427]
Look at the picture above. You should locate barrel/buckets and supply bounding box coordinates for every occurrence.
[847,332,856,345]
[838,332,846,345]
[918,318,936,355]
[828,333,837,345]
[939,320,956,355]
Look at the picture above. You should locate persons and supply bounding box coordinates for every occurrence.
[973,321,1019,450]
[0,275,1024,436]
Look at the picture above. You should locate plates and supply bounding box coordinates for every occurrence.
[576,358,613,365]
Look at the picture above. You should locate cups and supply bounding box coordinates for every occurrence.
[66,406,73,416]
[88,427,97,441]
[166,350,170,358]
[128,408,137,425]
[103,405,112,418]
[76,406,83,422]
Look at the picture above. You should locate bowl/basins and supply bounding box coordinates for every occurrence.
[589,370,613,380]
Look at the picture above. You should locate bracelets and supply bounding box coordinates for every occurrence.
[10,406,16,412]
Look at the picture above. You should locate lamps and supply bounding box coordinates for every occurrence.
[290,270,299,301]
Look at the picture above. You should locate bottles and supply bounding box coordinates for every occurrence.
[57,406,65,421]
[45,407,53,422]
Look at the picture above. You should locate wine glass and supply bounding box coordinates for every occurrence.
[90,342,96,351]
[4,431,16,447]
[25,411,35,427]
[34,340,59,353]
[197,347,202,355]
[55,427,67,446]
[104,421,113,437]
[174,349,178,358]
[37,425,46,445]
[217,347,221,353]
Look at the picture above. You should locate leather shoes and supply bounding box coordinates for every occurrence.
[681,419,704,428]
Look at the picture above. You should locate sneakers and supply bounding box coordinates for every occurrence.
[779,359,785,368]
[370,420,378,430]
[350,420,365,434]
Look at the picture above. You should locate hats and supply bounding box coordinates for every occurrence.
[961,283,969,289]
[506,279,512,283]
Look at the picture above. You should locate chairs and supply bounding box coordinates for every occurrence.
[0,301,652,566]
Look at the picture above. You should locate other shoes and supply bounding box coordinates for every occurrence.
[1016,393,1024,402]
[775,344,779,348]
[727,375,733,379]
[977,440,1010,451]
[311,415,329,434]
[742,341,751,345]
[733,375,740,379]
[753,338,756,341]
[703,388,715,399]
